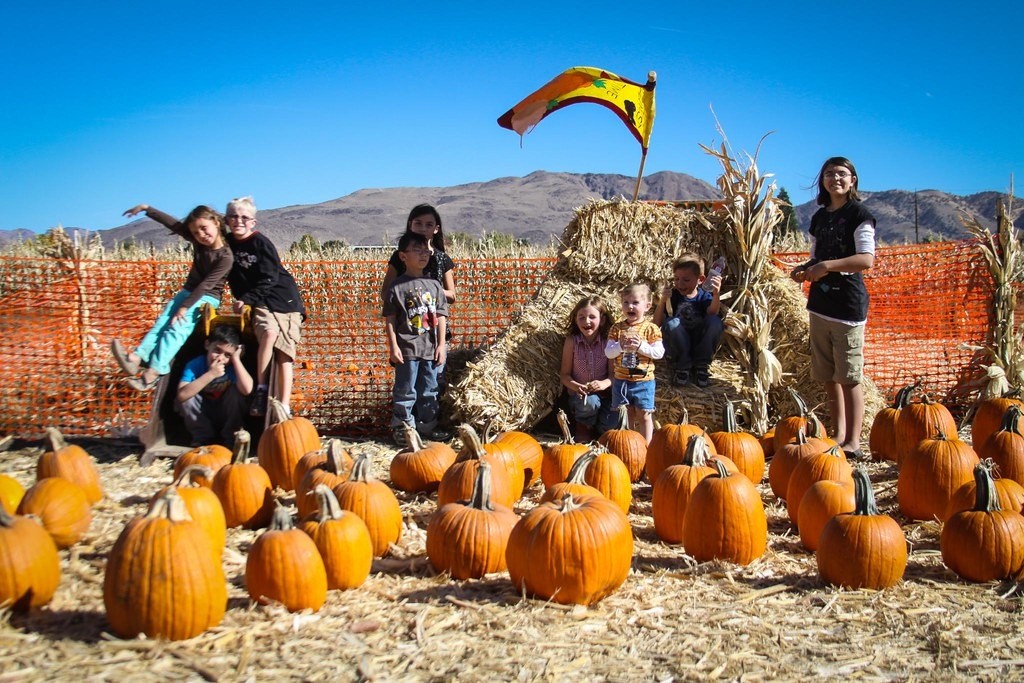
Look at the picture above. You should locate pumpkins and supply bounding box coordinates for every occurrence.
[1,380,1024,642]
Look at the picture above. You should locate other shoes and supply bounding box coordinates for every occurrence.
[844,448,863,459]
[112,338,138,375]
[393,428,408,446]
[421,426,451,441]
[130,376,161,391]
[673,370,691,385]
[694,369,708,388]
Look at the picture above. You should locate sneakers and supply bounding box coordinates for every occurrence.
[250,389,267,415]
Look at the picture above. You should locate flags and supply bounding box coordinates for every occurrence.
[496,67,656,154]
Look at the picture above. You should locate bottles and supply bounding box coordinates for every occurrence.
[621,326,637,369]
[701,267,722,292]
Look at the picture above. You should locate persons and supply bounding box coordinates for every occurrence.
[223,197,307,422]
[604,283,665,447]
[174,324,254,448]
[381,204,456,403]
[654,253,722,387]
[560,296,615,442]
[382,233,451,449]
[791,157,877,458]
[111,205,234,391]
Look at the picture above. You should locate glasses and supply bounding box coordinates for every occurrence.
[825,171,853,178]
[228,215,257,222]
[404,248,434,257]
